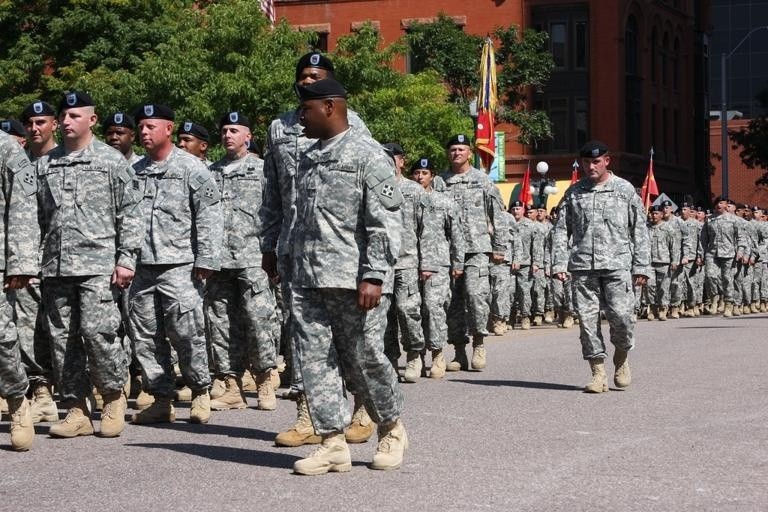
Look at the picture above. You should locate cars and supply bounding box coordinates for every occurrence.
[708,110,743,122]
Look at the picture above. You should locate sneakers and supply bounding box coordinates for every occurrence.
[48,399,95,438]
[638,294,768,322]
[583,357,610,393]
[0,396,34,451]
[613,348,632,388]
[487,311,579,336]
[23,386,59,423]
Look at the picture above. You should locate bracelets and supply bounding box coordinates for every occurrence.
[365,277,383,286]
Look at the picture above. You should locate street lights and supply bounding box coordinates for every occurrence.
[526,161,558,211]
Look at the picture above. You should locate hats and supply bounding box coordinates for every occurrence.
[647,194,768,213]
[578,139,609,157]
[510,200,557,214]
[296,51,335,78]
[134,104,174,127]
[219,112,251,131]
[57,90,96,111]
[0,101,55,137]
[384,143,432,173]
[102,112,134,130]
[293,78,347,100]
[446,134,470,149]
[176,121,208,142]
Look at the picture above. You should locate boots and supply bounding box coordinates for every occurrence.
[389,340,486,382]
[274,393,322,448]
[293,432,352,475]
[370,417,410,470]
[344,395,372,444]
[95,364,298,436]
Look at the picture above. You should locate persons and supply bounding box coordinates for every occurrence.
[288,75,410,478]
[550,138,651,393]
[253,48,376,449]
[509,195,767,330]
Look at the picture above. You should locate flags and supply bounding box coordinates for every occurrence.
[257,0,277,29]
[570,169,578,186]
[642,158,660,215]
[473,33,501,174]
[517,164,533,208]
